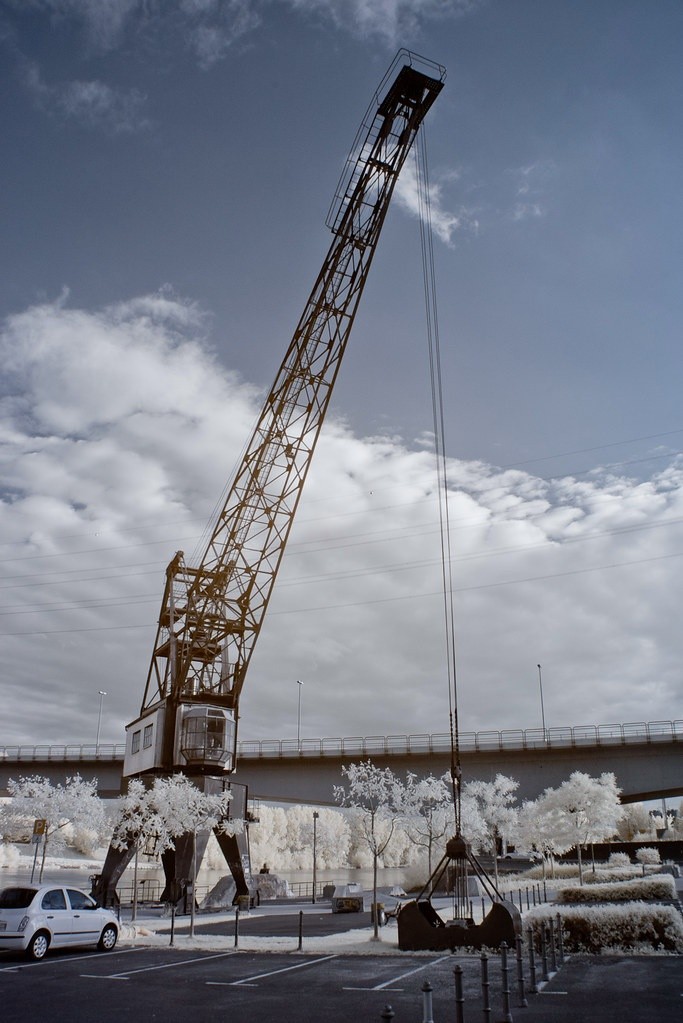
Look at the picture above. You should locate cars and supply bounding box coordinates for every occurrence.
[0,882,119,963]
[496,848,543,861]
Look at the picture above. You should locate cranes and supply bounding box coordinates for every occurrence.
[89,41,525,957]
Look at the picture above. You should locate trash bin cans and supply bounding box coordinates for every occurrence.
[239,895,250,911]
[370,903,385,926]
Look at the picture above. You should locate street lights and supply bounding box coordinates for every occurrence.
[96,691,107,756]
[297,680,303,751]
[537,664,546,742]
[312,811,319,905]
